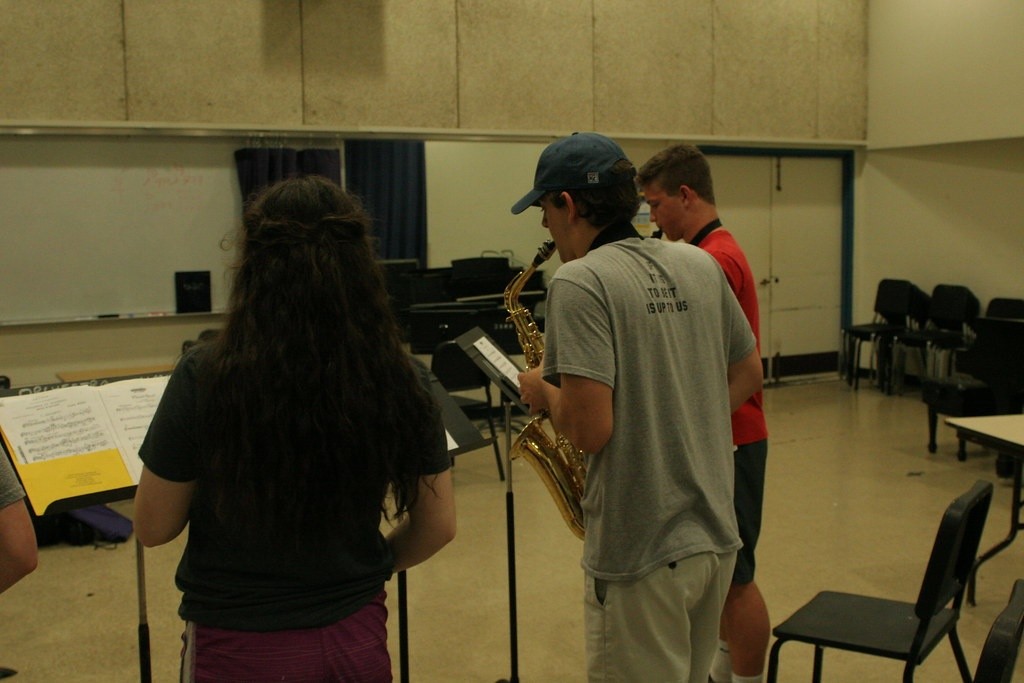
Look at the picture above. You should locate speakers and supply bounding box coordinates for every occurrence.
[390,255,547,353]
[174,271,212,314]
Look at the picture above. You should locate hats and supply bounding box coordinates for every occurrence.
[510,130,637,215]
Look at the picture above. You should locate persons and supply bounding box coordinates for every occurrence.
[0,443,39,596]
[635,143,771,683]
[133,174,458,683]
[510,131,764,683]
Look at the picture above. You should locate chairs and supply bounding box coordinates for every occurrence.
[841,278,981,395]
[767,479,995,682]
[920,297,1024,463]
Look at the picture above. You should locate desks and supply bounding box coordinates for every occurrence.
[941,413,1024,606]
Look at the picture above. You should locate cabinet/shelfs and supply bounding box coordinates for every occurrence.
[700,152,848,381]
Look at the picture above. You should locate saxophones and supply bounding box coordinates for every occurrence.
[503,237,589,541]
